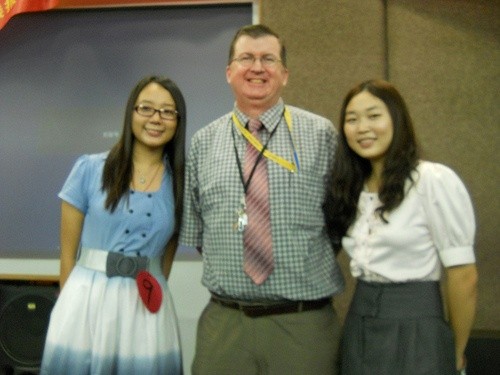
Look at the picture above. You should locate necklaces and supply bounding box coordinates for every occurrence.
[131,161,161,193]
[232,104,286,233]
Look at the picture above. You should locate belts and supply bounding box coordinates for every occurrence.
[80,247,162,279]
[212,291,334,319]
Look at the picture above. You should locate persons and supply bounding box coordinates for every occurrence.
[322,79,478,375]
[178,24,344,375]
[39,75,184,375]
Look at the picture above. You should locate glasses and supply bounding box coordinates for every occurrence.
[229,53,284,67]
[134,103,179,120]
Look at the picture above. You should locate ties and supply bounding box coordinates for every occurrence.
[241,120,275,286]
